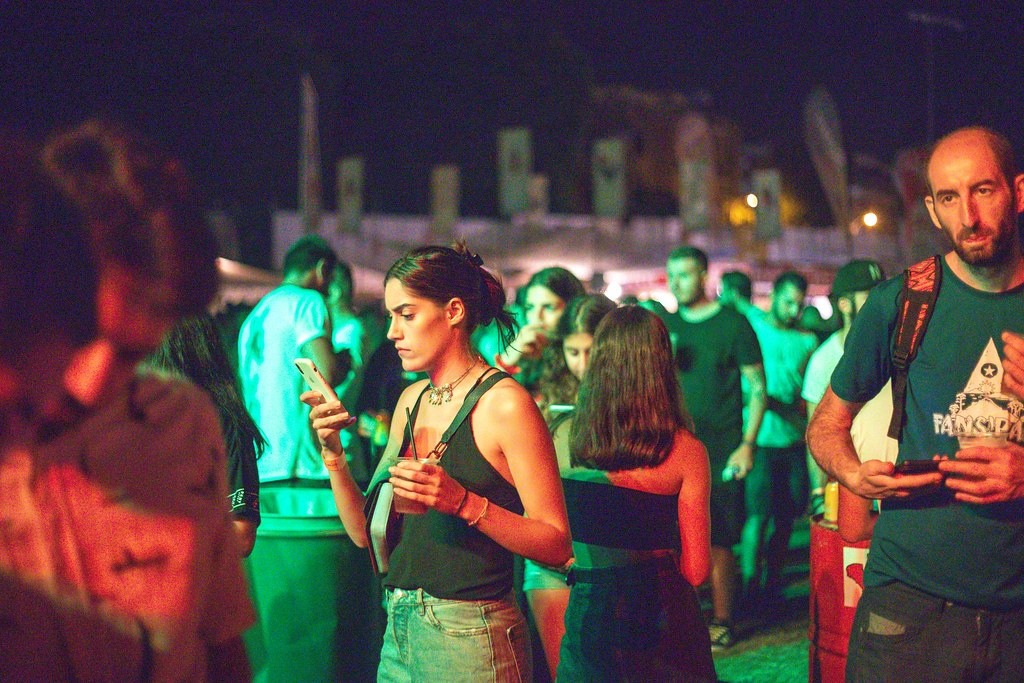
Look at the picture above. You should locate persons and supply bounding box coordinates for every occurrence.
[0,116,1023,683]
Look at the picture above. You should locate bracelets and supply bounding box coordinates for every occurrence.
[320,448,346,471]
[455,488,470,515]
[467,496,489,526]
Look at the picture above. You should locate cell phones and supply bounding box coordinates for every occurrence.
[548,405,576,421]
[294,359,351,424]
[894,459,946,477]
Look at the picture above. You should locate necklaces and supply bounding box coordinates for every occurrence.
[426,354,482,406]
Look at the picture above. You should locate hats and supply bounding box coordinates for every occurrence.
[831,259,887,297]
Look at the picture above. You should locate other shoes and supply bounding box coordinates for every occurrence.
[706,620,737,653]
